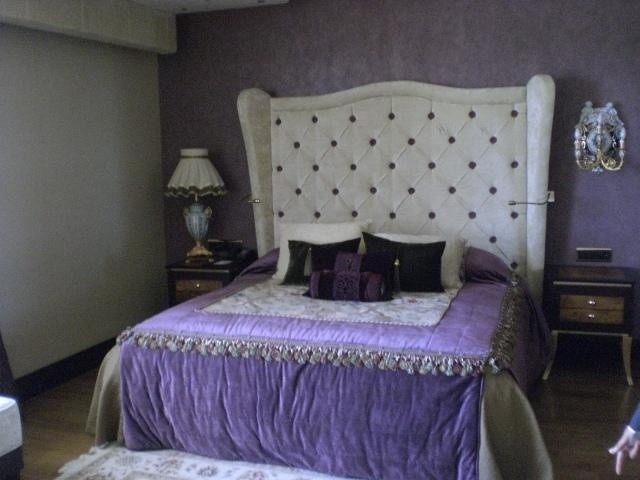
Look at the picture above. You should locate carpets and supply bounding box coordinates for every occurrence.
[51,439,354,479]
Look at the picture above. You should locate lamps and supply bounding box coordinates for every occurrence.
[164,147,231,266]
[572,98,627,173]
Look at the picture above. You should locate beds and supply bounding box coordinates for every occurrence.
[86,72,558,480]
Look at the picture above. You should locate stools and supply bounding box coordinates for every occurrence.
[1,387,24,480]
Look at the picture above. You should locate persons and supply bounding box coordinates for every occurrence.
[608,402,639,475]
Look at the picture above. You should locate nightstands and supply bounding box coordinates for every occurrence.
[540,266,636,387]
[166,249,256,304]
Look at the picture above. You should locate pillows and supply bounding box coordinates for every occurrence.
[268,219,465,306]
